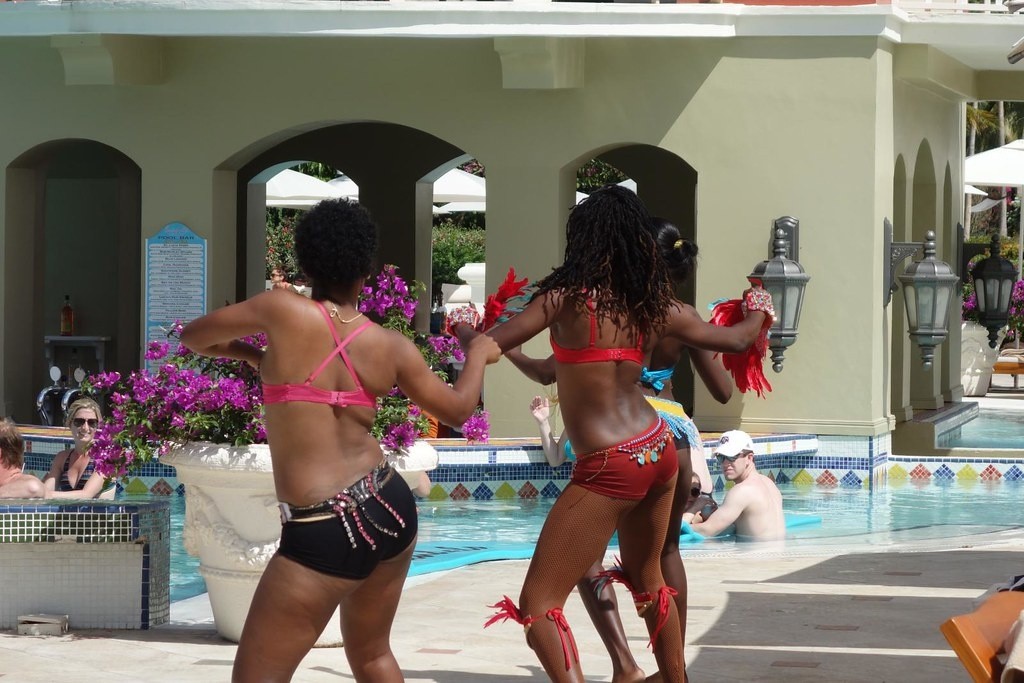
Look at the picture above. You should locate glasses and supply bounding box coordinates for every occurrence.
[718,450,753,463]
[690,488,702,498]
[71,418,98,428]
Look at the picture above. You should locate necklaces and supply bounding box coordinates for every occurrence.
[330,301,363,324]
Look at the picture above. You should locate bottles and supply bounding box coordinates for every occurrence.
[60,295,73,336]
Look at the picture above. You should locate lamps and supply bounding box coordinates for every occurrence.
[883,217,957,372]
[956,222,1020,349]
[746,215,812,373]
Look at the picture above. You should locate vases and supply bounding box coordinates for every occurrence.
[160,442,438,647]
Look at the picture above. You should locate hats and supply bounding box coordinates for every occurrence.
[714,430,753,457]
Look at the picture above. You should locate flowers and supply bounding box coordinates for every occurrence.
[76,263,489,483]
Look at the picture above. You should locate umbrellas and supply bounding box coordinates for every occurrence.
[965,138,1024,280]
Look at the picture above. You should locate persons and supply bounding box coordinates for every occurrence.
[413,471,432,498]
[42,395,116,501]
[447,184,784,683]
[0,417,50,499]
[270,268,299,294]
[180,199,500,683]
